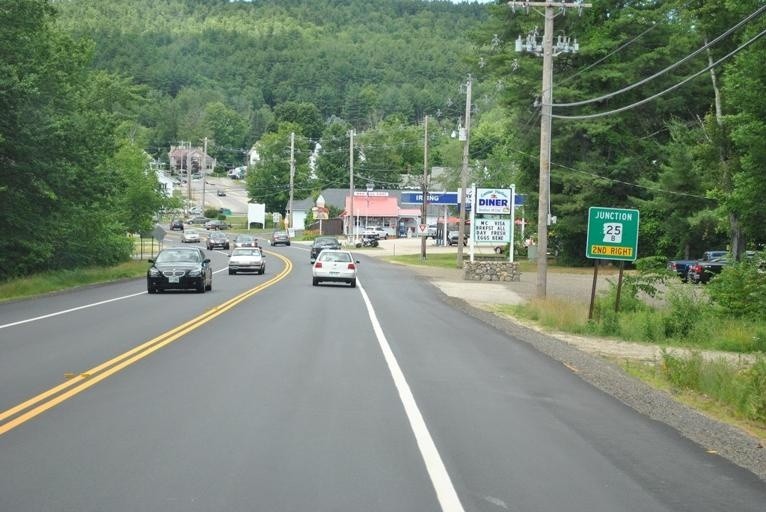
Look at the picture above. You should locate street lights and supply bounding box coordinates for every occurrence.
[449,127,470,269]
[363,183,375,229]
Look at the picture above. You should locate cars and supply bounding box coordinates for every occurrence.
[233,234,258,247]
[191,172,201,180]
[227,247,266,275]
[271,229,290,246]
[181,229,200,243]
[494,242,527,256]
[310,249,360,288]
[688,251,765,286]
[184,216,210,225]
[185,207,203,215]
[217,190,225,196]
[310,236,342,264]
[203,220,232,231]
[170,220,184,230]
[448,231,468,246]
[147,246,213,294]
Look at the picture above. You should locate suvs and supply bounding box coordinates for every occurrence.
[362,227,387,240]
[206,231,230,250]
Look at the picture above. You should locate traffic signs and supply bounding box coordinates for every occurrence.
[585,205,639,264]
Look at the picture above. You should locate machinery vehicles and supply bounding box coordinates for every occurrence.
[227,166,247,179]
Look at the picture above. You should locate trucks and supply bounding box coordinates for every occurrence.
[417,221,470,240]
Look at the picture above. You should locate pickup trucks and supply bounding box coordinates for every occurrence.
[667,251,733,284]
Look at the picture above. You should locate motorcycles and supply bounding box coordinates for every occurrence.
[355,231,380,248]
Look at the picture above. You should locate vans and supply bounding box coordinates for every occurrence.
[216,208,232,216]
